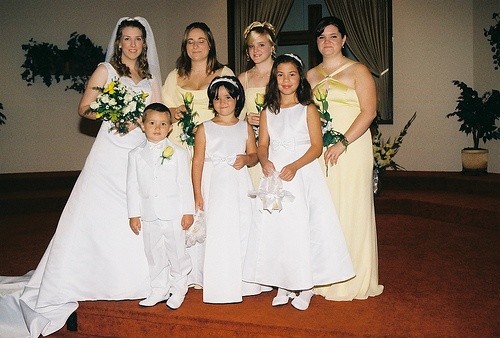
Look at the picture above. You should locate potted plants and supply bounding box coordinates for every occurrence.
[447,80,500,175]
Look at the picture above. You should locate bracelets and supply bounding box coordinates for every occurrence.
[339,137,350,147]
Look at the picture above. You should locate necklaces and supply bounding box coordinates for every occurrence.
[253,66,271,80]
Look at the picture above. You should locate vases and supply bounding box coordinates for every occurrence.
[373,168,379,193]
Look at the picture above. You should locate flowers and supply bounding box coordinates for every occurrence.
[255,93,265,113]
[83,76,149,134]
[372,111,416,173]
[174,92,199,145]
[314,88,348,177]
[160,146,174,164]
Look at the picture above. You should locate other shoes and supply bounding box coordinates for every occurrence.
[291,289,315,311]
[271,290,297,306]
[138,292,170,307]
[166,292,186,309]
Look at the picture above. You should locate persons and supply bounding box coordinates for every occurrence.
[79,16,377,311]
